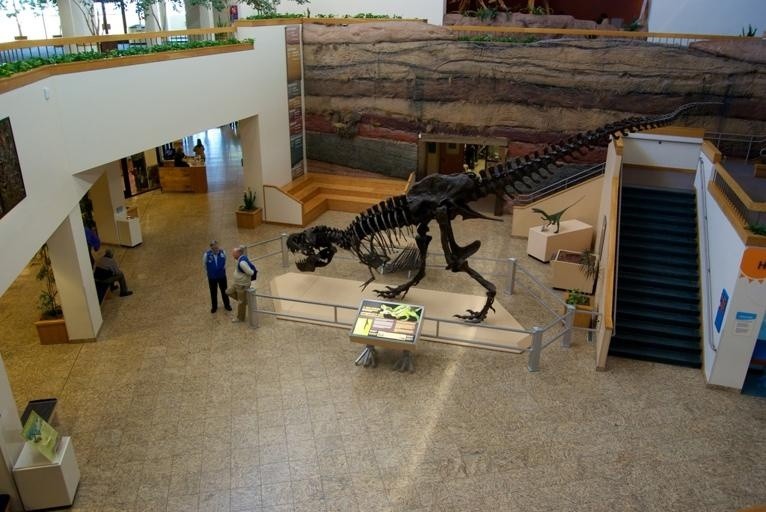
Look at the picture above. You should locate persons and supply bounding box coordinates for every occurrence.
[192,139,205,163]
[225,248,258,323]
[201,239,233,313]
[172,146,191,168]
[82,222,103,271]
[95,249,133,298]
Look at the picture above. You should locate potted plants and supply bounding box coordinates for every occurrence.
[553,247,600,295]
[35,241,69,345]
[564,288,594,329]
[235,186,262,229]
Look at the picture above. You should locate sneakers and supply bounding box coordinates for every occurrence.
[111,285,118,291]
[226,306,232,311]
[120,291,132,296]
[210,307,216,313]
[231,317,241,322]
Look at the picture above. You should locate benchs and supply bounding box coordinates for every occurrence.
[21,397,58,431]
[94,284,110,310]
